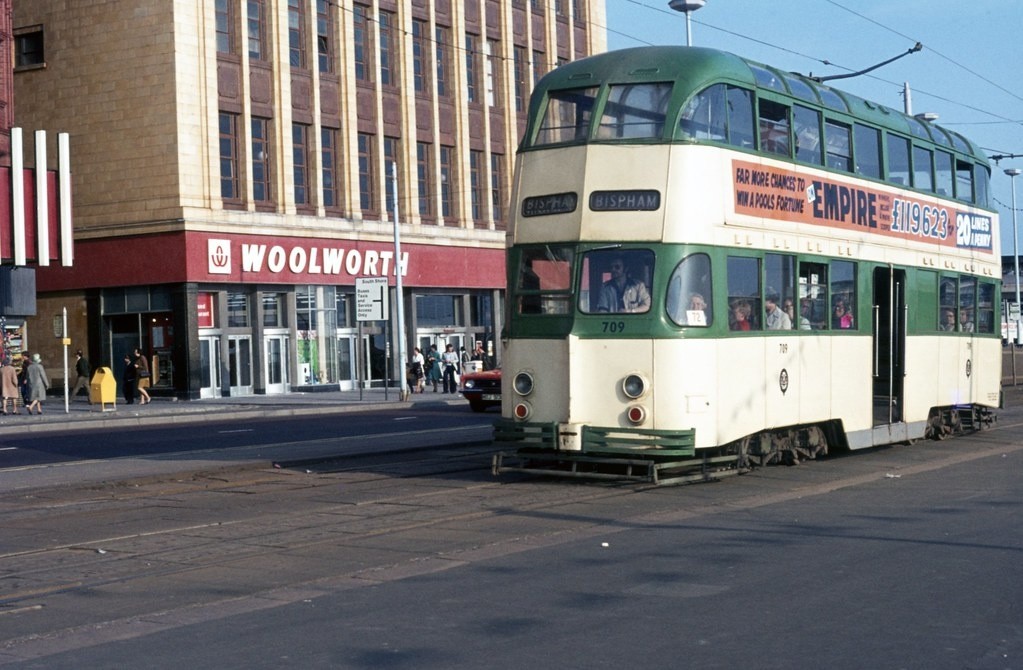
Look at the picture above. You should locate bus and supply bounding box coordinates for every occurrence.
[490,45,1007,487]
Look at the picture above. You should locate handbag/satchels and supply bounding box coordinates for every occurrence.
[139,366,150,378]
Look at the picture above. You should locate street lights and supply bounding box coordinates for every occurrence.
[669,0,707,46]
[1002,169,1023,347]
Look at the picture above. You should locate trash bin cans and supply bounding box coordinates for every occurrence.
[90,367,117,412]
[464,360,483,374]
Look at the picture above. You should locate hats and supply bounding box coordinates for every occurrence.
[32,354,41,363]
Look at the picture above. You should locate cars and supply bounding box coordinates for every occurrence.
[458,363,502,413]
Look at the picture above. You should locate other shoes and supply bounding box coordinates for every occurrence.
[138,402,144,405]
[37,412,42,415]
[26,405,30,410]
[27,407,32,415]
[146,397,152,403]
[13,412,21,415]
[3,412,8,415]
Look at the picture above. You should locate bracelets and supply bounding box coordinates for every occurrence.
[631,308,635,313]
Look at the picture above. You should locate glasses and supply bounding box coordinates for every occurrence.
[609,264,620,270]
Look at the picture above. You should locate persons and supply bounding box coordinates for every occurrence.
[597,257,650,314]
[122,355,137,405]
[682,292,707,325]
[728,288,854,331]
[0,357,20,415]
[68,350,92,405]
[943,307,974,332]
[26,354,49,415]
[18,351,32,407]
[412,344,493,394]
[133,347,151,405]
